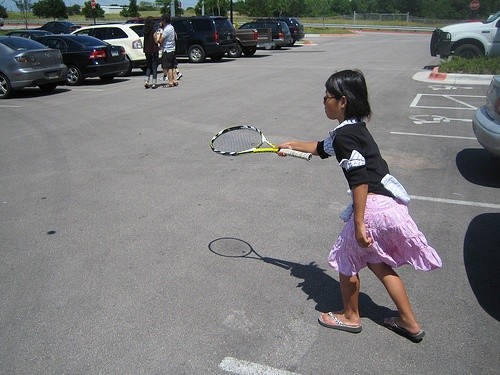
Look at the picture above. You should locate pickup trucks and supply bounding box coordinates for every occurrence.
[227,28,273,58]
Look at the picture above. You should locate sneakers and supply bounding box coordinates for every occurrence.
[176,73,182,80]
[159,76,168,81]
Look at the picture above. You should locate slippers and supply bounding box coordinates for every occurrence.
[383,316,426,341]
[317,311,362,333]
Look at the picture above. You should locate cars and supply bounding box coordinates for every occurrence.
[471,74,500,160]
[40,21,81,34]
[0,35,67,99]
[4,30,55,40]
[32,33,126,85]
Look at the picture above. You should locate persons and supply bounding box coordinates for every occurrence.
[273,69,442,341]
[143,17,159,88]
[158,14,183,87]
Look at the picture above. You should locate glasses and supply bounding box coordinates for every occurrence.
[324,95,337,101]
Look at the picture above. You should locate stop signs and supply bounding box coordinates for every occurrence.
[470,1,480,10]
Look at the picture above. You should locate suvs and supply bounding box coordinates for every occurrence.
[124,15,237,63]
[238,19,291,50]
[69,23,163,76]
[276,18,305,45]
[429,10,500,59]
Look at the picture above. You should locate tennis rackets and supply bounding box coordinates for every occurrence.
[210,124,312,161]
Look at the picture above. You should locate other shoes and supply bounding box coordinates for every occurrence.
[173,81,178,86]
[163,83,174,87]
[144,81,150,88]
[151,83,158,89]
